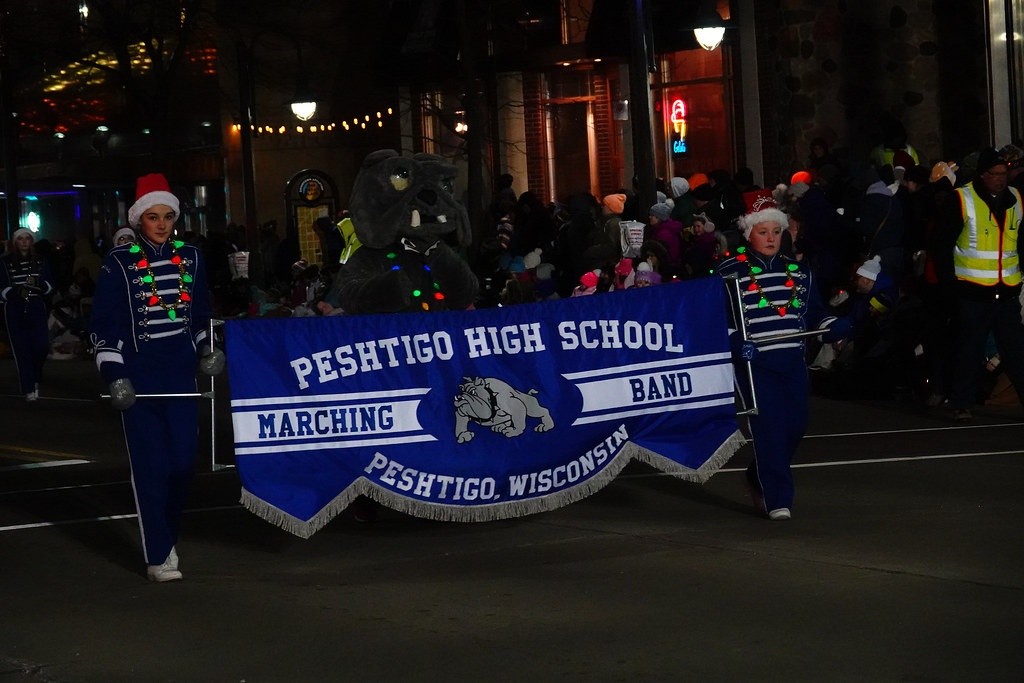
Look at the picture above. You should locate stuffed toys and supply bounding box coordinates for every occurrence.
[320,152,481,523]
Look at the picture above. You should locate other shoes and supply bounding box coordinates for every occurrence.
[26,392,36,402]
[948,405,973,422]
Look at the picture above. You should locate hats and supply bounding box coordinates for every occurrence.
[649,198,675,220]
[580,258,681,288]
[977,147,1007,175]
[603,193,627,214]
[292,258,319,279]
[693,183,715,200]
[112,227,136,247]
[737,188,790,231]
[537,262,555,280]
[524,248,542,270]
[128,173,180,229]
[856,255,882,281]
[509,256,525,273]
[689,172,708,191]
[13,228,34,248]
[904,164,931,183]
[671,176,688,198]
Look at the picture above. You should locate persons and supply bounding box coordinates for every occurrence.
[84,172,225,580]
[0,144,1023,420]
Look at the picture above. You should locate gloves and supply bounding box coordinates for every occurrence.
[200,344,226,376]
[27,276,42,289]
[16,285,30,300]
[109,378,136,410]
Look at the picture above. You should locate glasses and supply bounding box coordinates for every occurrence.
[986,170,1009,176]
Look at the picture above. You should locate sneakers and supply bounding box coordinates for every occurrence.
[770,508,791,521]
[146,545,183,582]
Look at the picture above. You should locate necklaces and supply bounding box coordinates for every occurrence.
[737,245,801,317]
[130,237,194,321]
